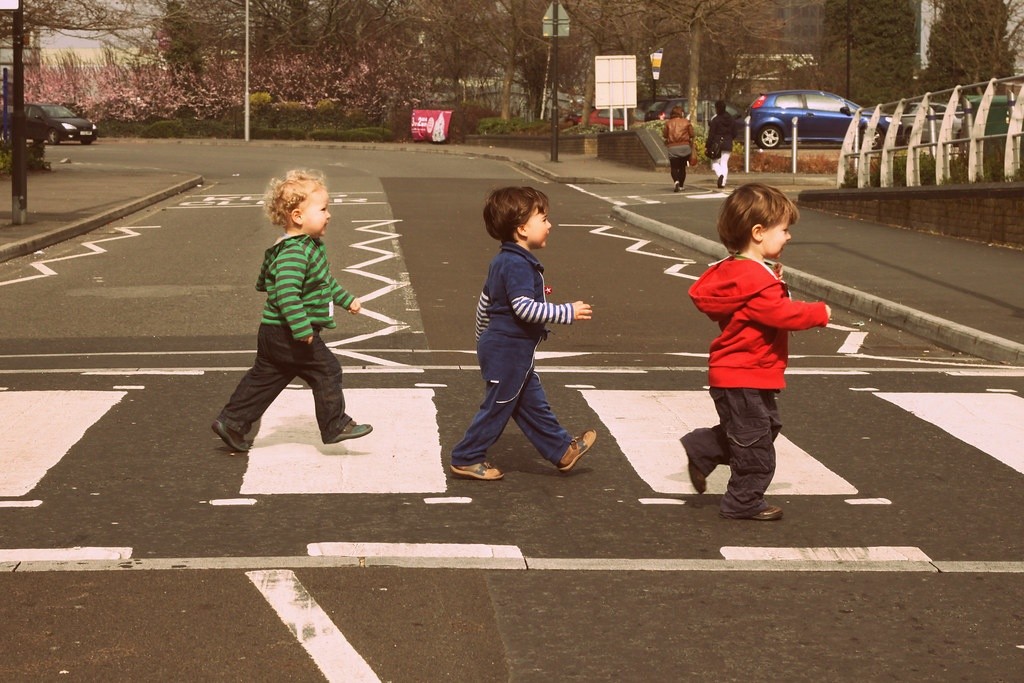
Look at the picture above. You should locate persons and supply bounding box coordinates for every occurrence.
[705,101,737,188]
[212,169,373,452]
[678,183,832,521]
[663,106,695,192]
[451,187,597,480]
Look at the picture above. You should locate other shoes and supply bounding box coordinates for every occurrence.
[212,419,248,452]
[718,175,723,188]
[674,181,679,192]
[722,186,725,188]
[680,187,685,191]
[687,453,706,494]
[748,506,784,521]
[324,420,373,444]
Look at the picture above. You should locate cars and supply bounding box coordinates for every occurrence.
[564,106,644,129]
[644,97,745,131]
[0,102,99,146]
[891,98,964,148]
[741,89,904,151]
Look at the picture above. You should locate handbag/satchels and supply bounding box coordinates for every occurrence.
[689,144,698,166]
[704,142,721,160]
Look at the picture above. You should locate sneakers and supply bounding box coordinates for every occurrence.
[556,429,596,472]
[450,461,504,480]
